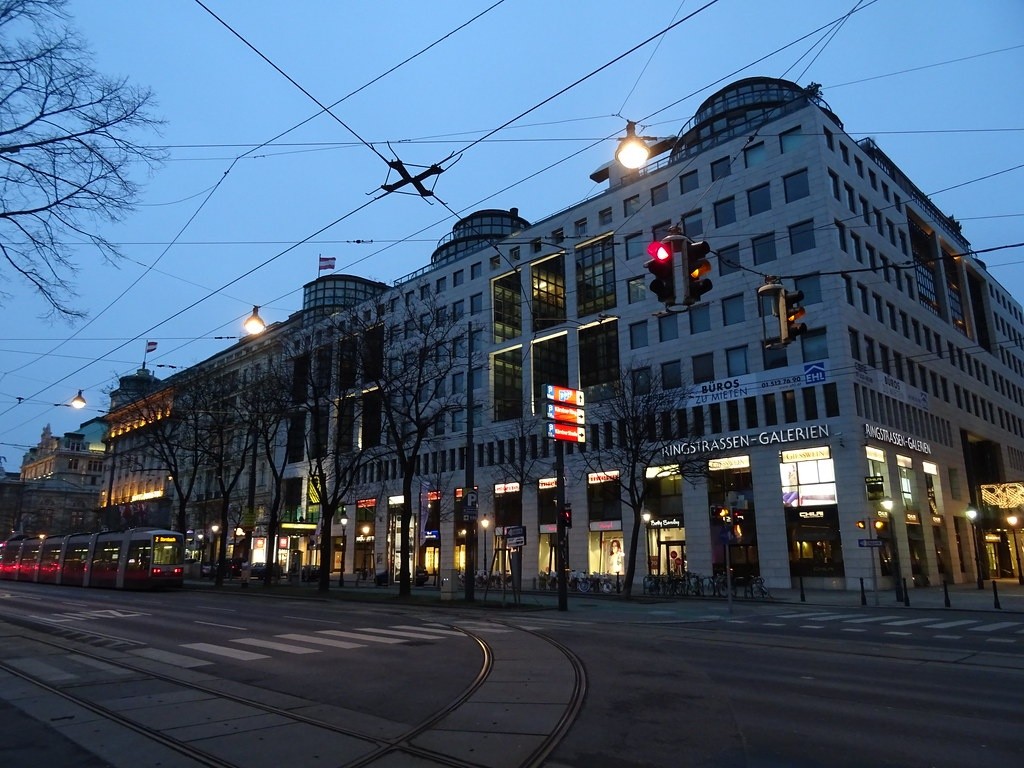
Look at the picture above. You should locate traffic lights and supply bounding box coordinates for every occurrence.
[710,507,725,518]
[647,240,674,305]
[681,239,713,302]
[732,506,739,525]
[564,508,573,529]
[778,288,807,342]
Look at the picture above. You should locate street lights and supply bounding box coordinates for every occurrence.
[362,524,371,580]
[880,496,904,603]
[641,509,653,575]
[964,502,984,590]
[1006,509,1024,586]
[481,513,489,575]
[338,514,349,587]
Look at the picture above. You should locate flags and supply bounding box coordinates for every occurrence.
[147,342,158,353]
[319,257,336,270]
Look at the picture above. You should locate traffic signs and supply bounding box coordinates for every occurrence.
[542,385,585,443]
[506,526,524,538]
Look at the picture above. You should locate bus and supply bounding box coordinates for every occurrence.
[0,528,185,592]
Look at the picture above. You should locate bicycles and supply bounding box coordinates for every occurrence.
[456,568,769,600]
[299,564,321,581]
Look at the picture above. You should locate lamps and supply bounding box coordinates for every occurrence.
[243,306,265,335]
[70,390,87,409]
[614,122,651,170]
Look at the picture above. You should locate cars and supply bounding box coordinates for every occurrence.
[249,562,267,577]
[201,558,244,579]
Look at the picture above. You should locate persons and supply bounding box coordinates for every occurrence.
[608,540,625,575]
